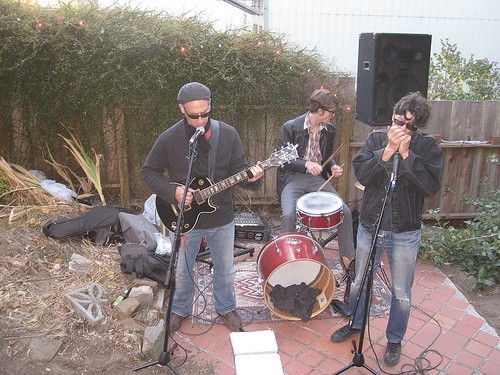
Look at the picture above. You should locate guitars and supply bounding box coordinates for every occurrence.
[155,142,301,234]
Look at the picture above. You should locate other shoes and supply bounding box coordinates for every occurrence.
[222,312,241,330]
[163,312,183,331]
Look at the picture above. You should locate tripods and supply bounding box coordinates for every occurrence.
[133,141,199,375]
[331,173,393,375]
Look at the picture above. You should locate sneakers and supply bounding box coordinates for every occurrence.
[383,341,401,367]
[330,325,359,341]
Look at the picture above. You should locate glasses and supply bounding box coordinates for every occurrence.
[394,114,418,131]
[324,108,336,116]
[183,105,212,119]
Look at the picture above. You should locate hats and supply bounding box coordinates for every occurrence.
[177,83,211,103]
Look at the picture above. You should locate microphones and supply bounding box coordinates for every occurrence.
[391,152,400,189]
[189,126,205,144]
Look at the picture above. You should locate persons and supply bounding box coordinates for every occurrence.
[140,82,264,332]
[331,91,444,367]
[276,87,356,275]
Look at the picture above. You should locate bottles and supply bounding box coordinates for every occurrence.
[111,288,129,308]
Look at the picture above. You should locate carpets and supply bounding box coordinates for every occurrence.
[192,254,395,326]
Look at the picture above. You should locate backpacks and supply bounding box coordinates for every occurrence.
[120,255,174,287]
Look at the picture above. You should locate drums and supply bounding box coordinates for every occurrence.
[256,231,336,321]
[295,191,345,229]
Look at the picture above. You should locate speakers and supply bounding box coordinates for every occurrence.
[354,32,433,126]
